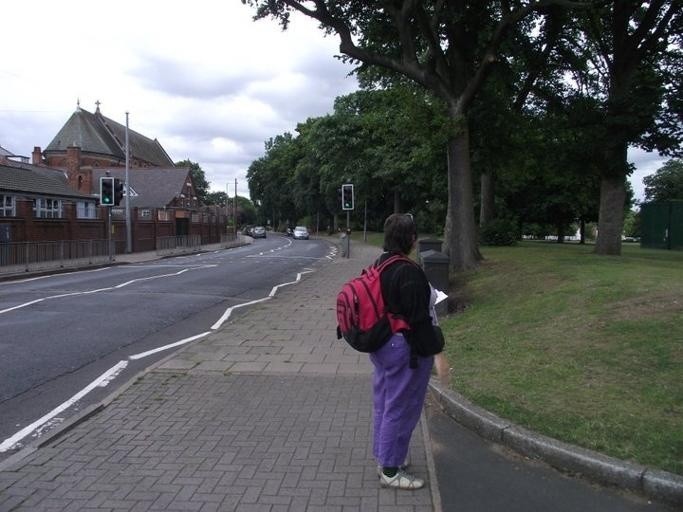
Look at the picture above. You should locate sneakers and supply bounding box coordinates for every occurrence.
[376,457,409,478]
[379,468,425,490]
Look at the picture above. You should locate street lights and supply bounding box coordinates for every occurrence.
[225,182,239,216]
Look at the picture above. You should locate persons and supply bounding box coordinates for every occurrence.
[362,212,450,492]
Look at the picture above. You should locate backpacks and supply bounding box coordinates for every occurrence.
[336,255,430,370]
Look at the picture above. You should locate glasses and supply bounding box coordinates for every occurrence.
[405,213,414,224]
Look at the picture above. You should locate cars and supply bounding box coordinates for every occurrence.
[241,223,313,241]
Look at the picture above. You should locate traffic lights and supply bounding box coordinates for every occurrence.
[114,179,124,207]
[100,177,114,206]
[336,185,342,204]
[342,183,355,210]
